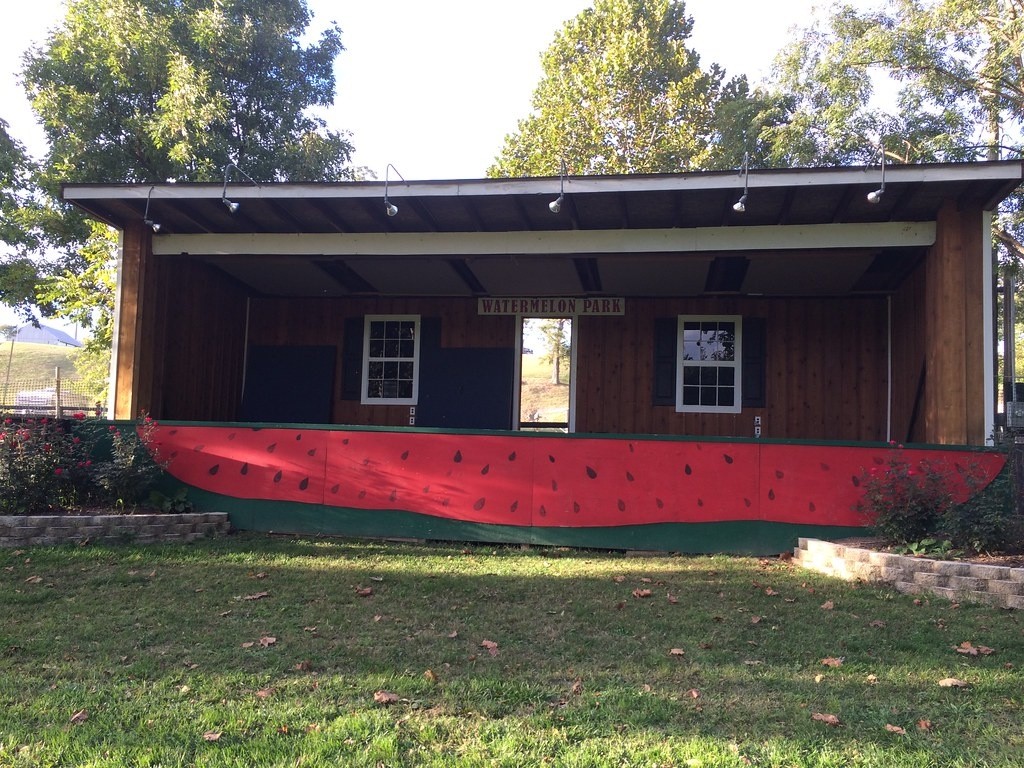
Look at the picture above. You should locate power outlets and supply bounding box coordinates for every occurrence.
[755,416,761,425]
[755,427,761,435]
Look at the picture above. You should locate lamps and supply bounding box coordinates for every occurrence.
[222,164,262,213]
[732,151,749,212]
[383,163,409,217]
[549,158,572,214]
[865,144,885,204]
[144,187,162,233]
